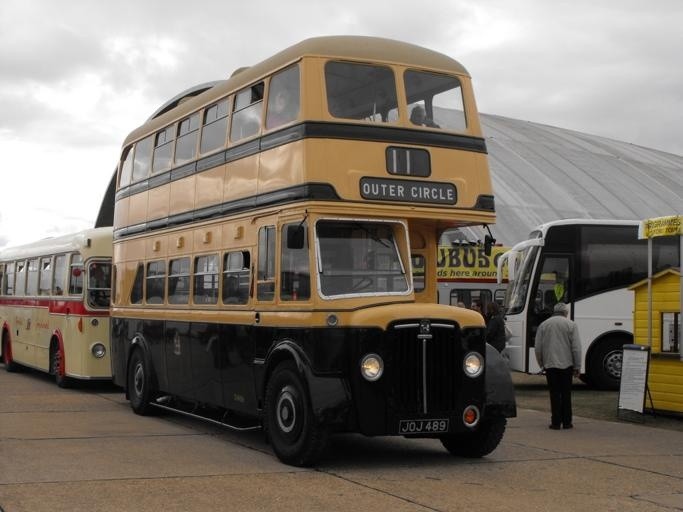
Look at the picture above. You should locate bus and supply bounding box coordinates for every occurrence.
[0,226,114,388]
[114,34,517,467]
[495,219,682,391]
[361,241,516,319]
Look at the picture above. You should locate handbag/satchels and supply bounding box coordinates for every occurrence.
[503,318,513,341]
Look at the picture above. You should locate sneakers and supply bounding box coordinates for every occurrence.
[563,423,572,428]
[549,425,560,429]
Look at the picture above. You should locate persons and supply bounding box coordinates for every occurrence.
[485,303,505,354]
[456,301,465,308]
[410,107,440,128]
[534,302,582,429]
[471,300,486,322]
